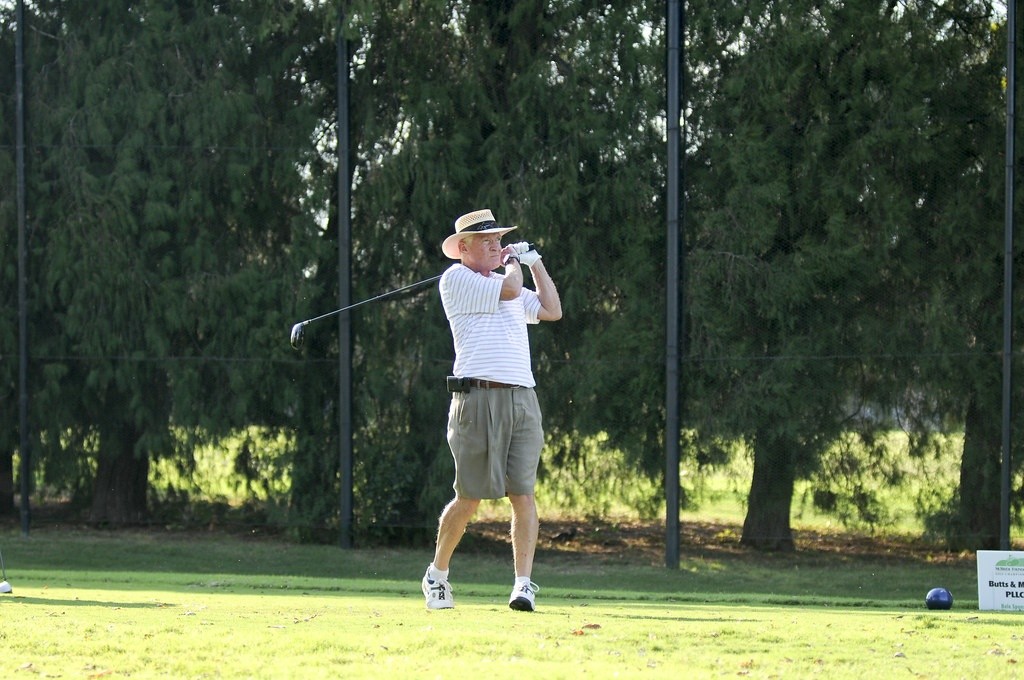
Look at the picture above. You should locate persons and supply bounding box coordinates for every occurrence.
[422,210,562,611]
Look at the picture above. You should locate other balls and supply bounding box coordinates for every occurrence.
[925,587,953,610]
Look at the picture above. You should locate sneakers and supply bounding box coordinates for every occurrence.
[422,566,454,608]
[509,581,539,611]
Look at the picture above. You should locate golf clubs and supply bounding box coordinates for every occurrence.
[290,242,535,352]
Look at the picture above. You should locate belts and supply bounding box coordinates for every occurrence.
[471,379,520,388]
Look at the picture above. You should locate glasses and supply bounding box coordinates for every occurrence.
[468,235,501,244]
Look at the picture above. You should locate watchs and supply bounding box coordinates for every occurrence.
[503,254,519,265]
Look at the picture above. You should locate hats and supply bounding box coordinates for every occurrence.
[442,209,518,259]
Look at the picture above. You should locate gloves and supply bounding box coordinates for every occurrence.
[509,242,542,268]
